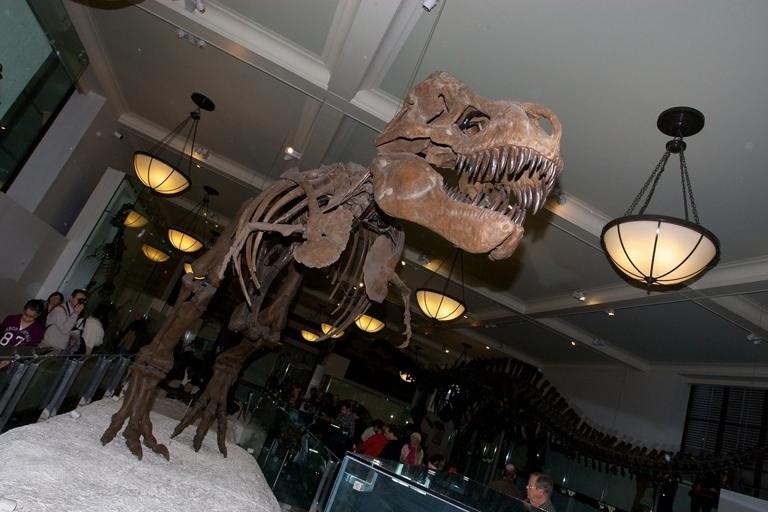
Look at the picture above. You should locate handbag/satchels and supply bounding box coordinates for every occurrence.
[67,326,88,361]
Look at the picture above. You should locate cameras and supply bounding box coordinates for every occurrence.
[78,298,88,305]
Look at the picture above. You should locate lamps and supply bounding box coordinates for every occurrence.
[167,184,218,255]
[598,105,720,291]
[300,307,321,341]
[355,313,386,332]
[412,245,468,324]
[132,90,216,199]
[139,242,170,263]
[121,208,149,229]
[321,312,345,339]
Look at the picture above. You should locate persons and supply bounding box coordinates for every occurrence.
[400,432,424,465]
[273,377,361,438]
[73,305,105,376]
[41,291,64,326]
[16,287,88,409]
[526,471,554,511]
[108,313,237,413]
[0,298,46,395]
[353,420,401,462]
[489,464,519,498]
